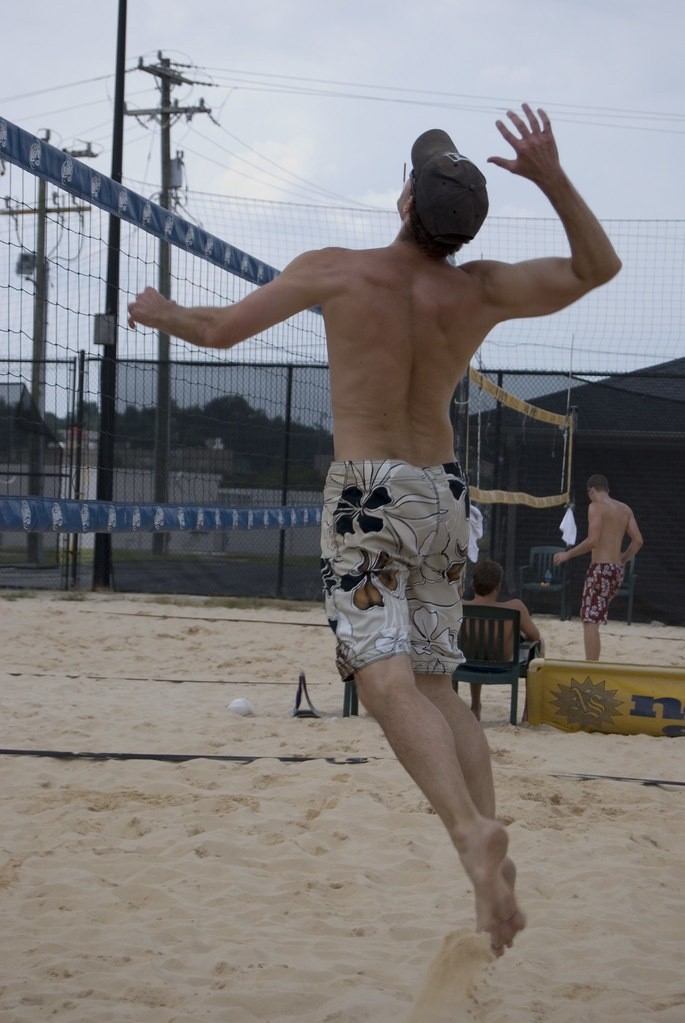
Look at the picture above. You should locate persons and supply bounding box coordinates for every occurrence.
[124,101,624,959]
[460,558,545,722]
[552,473,644,661]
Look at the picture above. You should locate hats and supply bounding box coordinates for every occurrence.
[411,129,490,244]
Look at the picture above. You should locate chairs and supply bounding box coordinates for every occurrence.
[609,554,637,625]
[453,605,541,726]
[519,545,578,621]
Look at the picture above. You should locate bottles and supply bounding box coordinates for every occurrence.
[545,569,552,583]
